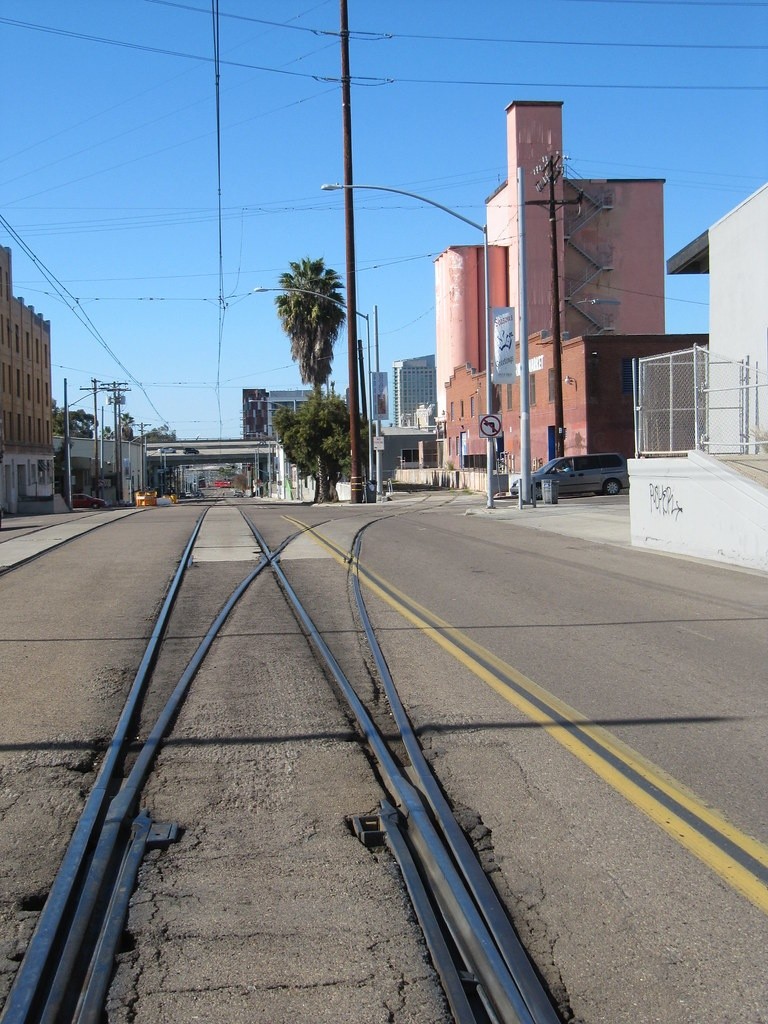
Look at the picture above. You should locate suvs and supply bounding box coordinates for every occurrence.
[183,447,199,454]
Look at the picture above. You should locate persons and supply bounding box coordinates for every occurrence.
[559,462,571,472]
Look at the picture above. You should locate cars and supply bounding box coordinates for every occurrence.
[234,491,244,497]
[180,492,201,498]
[158,447,176,453]
[65,494,107,509]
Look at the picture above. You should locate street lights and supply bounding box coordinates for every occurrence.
[248,399,299,499]
[320,184,494,508]
[254,287,373,482]
[64,377,109,509]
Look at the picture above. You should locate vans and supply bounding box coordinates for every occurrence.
[510,452,629,500]
